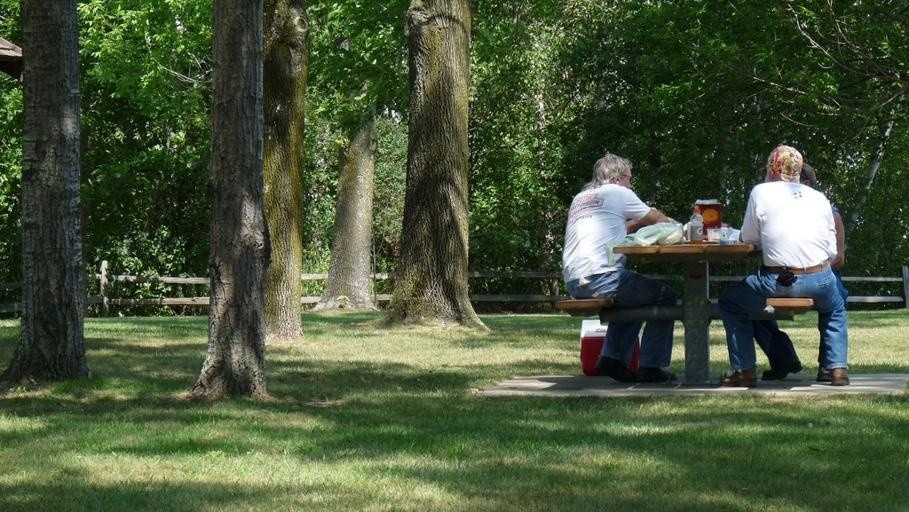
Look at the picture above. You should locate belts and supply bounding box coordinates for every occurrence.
[759,262,830,275]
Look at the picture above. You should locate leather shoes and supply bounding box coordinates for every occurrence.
[761,360,803,380]
[595,356,678,382]
[721,368,756,387]
[816,368,850,385]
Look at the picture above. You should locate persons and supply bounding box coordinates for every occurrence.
[722,146,849,386]
[562,154,678,383]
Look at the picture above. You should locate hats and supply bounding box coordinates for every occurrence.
[766,145,804,183]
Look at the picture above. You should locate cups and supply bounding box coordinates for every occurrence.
[705,227,722,244]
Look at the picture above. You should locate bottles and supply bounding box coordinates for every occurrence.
[689,203,705,244]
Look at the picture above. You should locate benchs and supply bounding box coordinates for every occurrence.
[555,279,817,388]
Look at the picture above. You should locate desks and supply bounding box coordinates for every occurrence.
[617,234,759,388]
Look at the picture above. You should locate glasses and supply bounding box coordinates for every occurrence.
[611,174,632,185]
